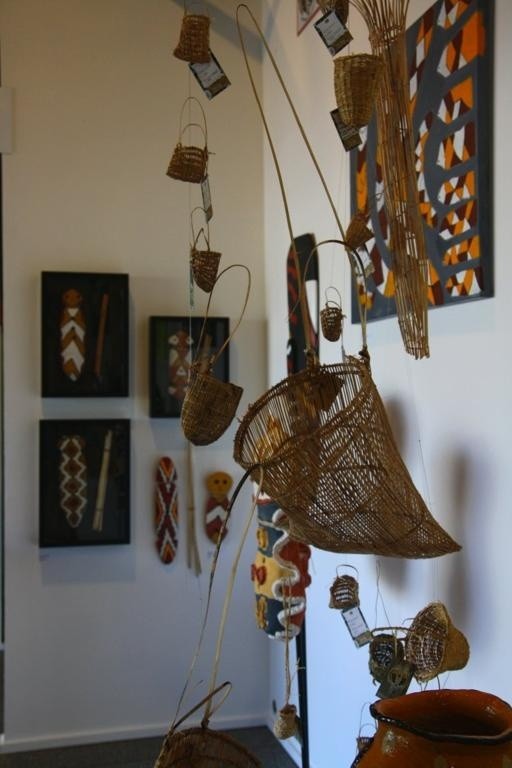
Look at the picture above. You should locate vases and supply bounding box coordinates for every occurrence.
[343,690,512,767]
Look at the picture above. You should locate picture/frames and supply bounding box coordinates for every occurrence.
[34,265,135,402]
[146,309,235,424]
[33,414,136,552]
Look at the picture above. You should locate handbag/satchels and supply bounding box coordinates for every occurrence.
[165,96,213,186]
[361,598,470,701]
[318,285,347,345]
[327,561,362,613]
[178,369,248,448]
[171,2,214,65]
[356,722,379,755]
[229,359,464,560]
[189,210,223,295]
[151,680,267,768]
[270,701,301,742]
[330,52,387,132]
[343,211,376,253]
